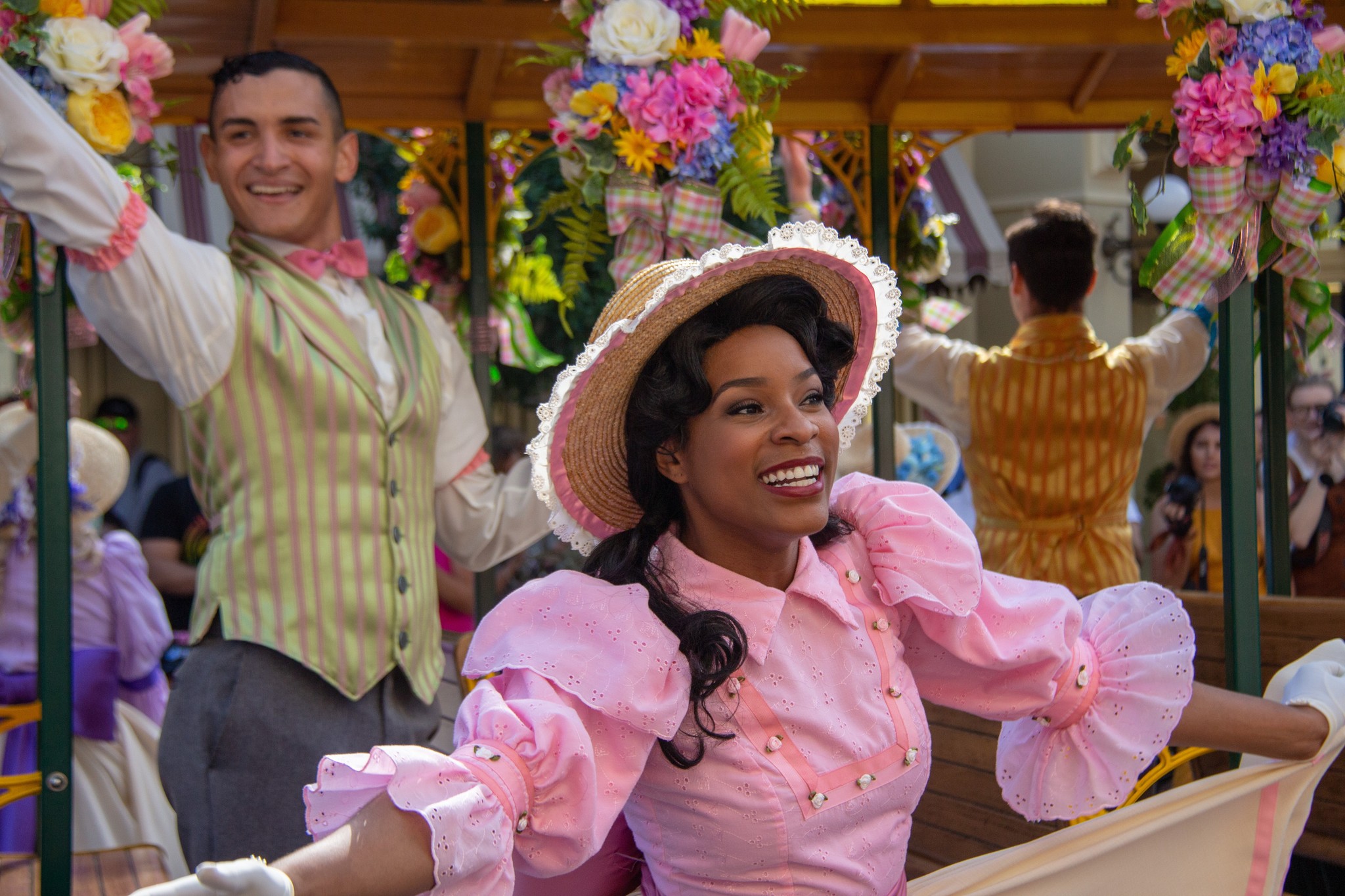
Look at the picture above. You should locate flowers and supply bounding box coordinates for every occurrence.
[0,0,186,433]
[386,0,962,383]
[1112,0,1345,379]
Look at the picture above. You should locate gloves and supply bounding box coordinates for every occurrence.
[127,855,295,896]
[1280,661,1345,763]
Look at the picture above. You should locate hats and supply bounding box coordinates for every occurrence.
[1165,403,1220,471]
[831,420,961,498]
[0,401,131,538]
[524,220,903,558]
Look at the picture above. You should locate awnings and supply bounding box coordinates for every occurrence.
[144,126,400,275]
[925,131,1012,299]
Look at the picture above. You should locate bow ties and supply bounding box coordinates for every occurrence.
[286,239,369,279]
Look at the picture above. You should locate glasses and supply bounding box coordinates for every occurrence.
[1292,405,1327,422]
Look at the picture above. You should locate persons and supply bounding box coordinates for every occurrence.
[778,130,1220,604]
[130,224,1345,896]
[0,390,594,896]
[890,418,977,532]
[1120,375,1345,603]
[0,46,558,877]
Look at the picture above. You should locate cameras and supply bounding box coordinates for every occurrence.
[1167,476,1202,514]
